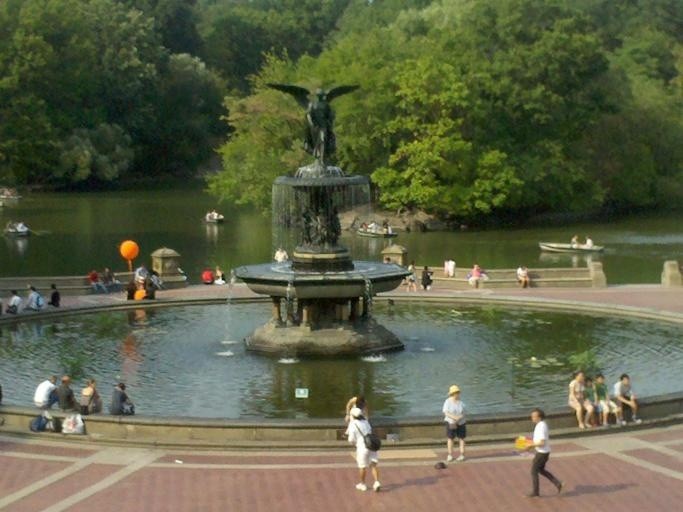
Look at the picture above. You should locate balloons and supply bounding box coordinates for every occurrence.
[118,240,139,261]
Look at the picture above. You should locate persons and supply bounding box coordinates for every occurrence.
[55,375,78,412]
[566,369,643,430]
[382,256,485,293]
[31,375,58,410]
[273,245,288,263]
[441,385,468,460]
[6,219,28,233]
[101,266,120,292]
[515,263,530,288]
[305,89,335,168]
[570,235,579,248]
[5,290,21,313]
[87,268,108,294]
[213,264,225,284]
[345,397,370,451]
[358,217,393,236]
[201,266,214,284]
[47,284,59,306]
[127,262,165,300]
[108,382,135,416]
[523,408,564,496]
[23,286,42,311]
[585,235,592,248]
[78,379,103,414]
[347,407,381,492]
[205,208,219,222]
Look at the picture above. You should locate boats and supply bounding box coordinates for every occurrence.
[538,242,605,254]
[205,212,225,222]
[7,230,32,236]
[357,229,398,238]
[2,196,23,201]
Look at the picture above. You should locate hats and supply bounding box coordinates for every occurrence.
[447,384,461,395]
[351,408,363,417]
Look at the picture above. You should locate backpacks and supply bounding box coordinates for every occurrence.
[36,295,44,307]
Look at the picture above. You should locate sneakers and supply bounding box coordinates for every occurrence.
[447,454,453,462]
[356,483,368,491]
[372,481,381,492]
[456,455,464,461]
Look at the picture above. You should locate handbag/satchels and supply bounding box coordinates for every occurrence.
[29,415,61,432]
[81,405,88,415]
[8,305,18,314]
[364,433,380,451]
